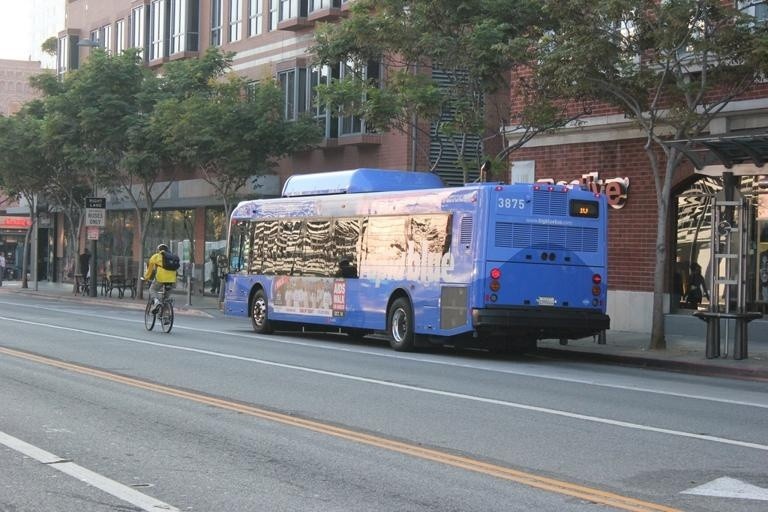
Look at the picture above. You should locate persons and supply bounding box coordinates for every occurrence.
[0,251,6,281]
[684,262,709,308]
[80,249,92,291]
[141,244,178,325]
[210,257,220,294]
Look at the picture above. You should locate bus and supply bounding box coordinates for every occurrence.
[216,167,610,353]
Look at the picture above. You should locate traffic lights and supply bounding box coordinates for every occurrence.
[37,191,51,218]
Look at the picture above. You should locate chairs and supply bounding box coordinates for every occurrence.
[75,272,137,299]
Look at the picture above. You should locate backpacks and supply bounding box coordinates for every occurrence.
[162,252,180,270]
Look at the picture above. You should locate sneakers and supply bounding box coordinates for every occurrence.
[152,301,162,313]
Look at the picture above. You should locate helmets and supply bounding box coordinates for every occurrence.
[159,245,168,251]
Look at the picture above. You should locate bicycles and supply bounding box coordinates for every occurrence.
[140,276,174,333]
